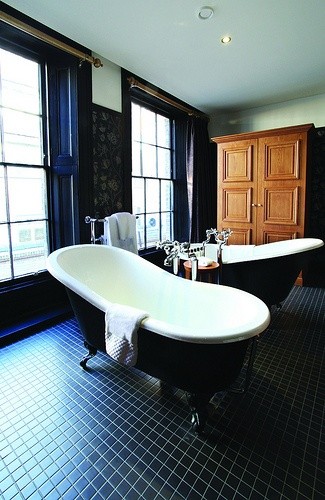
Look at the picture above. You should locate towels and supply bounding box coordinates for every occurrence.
[104,212,138,255]
[105,303,149,367]
[196,256,212,267]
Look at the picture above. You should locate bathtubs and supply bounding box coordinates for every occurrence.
[45,244,270,397]
[177,238,324,306]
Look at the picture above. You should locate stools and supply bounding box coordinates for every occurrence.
[184,257,220,284]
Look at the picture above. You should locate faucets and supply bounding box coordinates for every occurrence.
[164,252,177,266]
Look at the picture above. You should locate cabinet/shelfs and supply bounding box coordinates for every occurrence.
[210,123,325,287]
